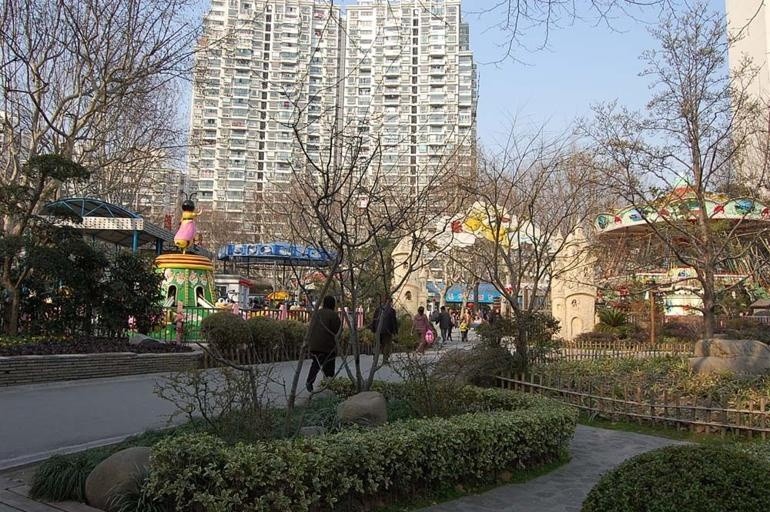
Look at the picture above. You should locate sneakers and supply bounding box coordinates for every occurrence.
[306,383,313,392]
[443,337,469,345]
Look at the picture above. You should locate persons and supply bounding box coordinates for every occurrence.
[372,297,398,365]
[409,303,503,356]
[306,296,341,392]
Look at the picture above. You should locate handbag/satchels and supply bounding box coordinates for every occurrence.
[425,329,435,344]
